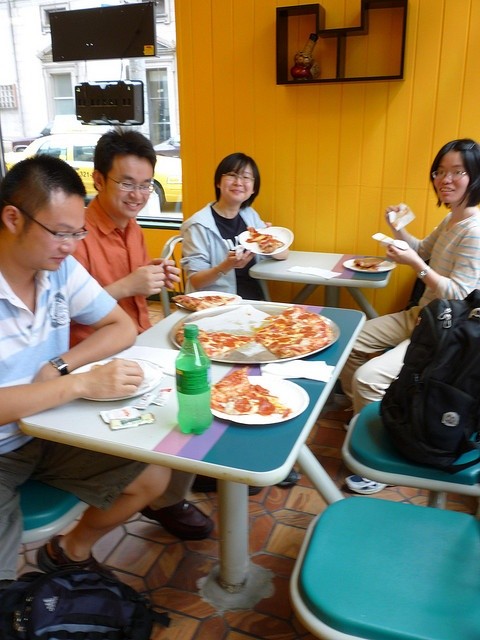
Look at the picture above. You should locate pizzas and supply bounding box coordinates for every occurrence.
[171,295,236,312]
[255,305,334,360]
[354,259,383,267]
[249,227,283,254]
[210,368,293,418]
[176,323,255,357]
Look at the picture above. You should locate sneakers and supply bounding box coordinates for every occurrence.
[37,535,120,582]
[345,475,396,494]
[344,416,356,430]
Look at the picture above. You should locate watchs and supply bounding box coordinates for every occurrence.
[417,265,431,279]
[49,356,70,377]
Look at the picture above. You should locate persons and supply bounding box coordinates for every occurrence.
[69,129,216,545]
[338,138,479,495]
[179,152,303,499]
[0,154,173,582]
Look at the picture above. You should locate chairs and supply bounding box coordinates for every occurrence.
[157,235,186,319]
[18,298,369,595]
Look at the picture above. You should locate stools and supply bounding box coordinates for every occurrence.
[19,477,89,543]
[290,495,480,640]
[343,400,480,510]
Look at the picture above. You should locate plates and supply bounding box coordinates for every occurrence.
[168,300,343,367]
[203,374,311,427]
[175,290,243,314]
[342,257,398,275]
[65,356,163,402]
[237,225,295,257]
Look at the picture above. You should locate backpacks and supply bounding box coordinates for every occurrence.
[1,572,173,639]
[380,289,480,474]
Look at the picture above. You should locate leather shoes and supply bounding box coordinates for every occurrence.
[139,500,214,539]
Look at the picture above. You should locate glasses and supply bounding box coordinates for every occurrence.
[220,172,255,182]
[0,197,88,240]
[430,171,468,179]
[101,173,156,194]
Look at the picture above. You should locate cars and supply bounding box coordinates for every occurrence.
[0,134,182,204]
[13,138,35,151]
[154,137,181,156]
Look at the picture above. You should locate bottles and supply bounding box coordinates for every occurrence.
[173,322,215,437]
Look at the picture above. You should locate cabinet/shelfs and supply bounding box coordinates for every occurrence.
[267,1,405,86]
